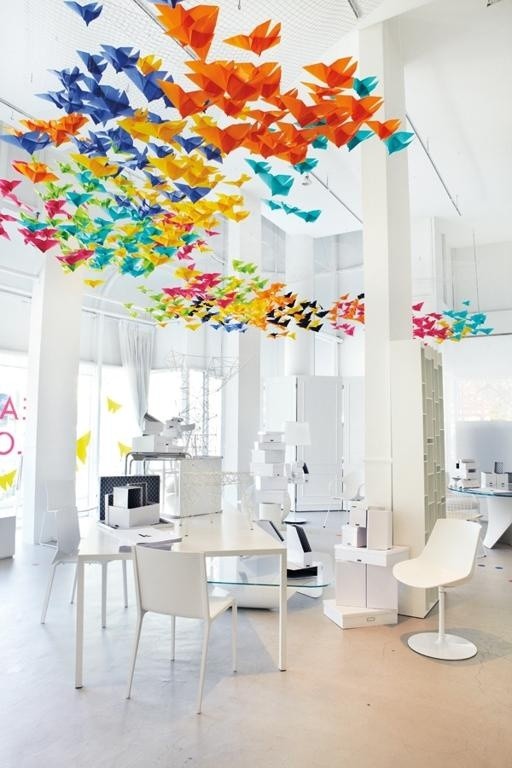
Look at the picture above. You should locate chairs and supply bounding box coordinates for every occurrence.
[393,517,483,661]
[42,506,132,632]
[124,544,236,716]
[323,457,364,528]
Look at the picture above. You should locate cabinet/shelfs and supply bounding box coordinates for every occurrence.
[125,452,223,518]
[322,544,410,630]
[392,339,447,618]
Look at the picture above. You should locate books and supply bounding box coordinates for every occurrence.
[469,486,512,496]
[116,524,182,546]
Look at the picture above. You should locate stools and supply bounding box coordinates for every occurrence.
[1,515,17,560]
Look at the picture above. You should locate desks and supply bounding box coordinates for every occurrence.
[76,511,290,688]
[447,485,511,549]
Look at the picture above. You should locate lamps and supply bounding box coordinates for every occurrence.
[283,422,312,476]
